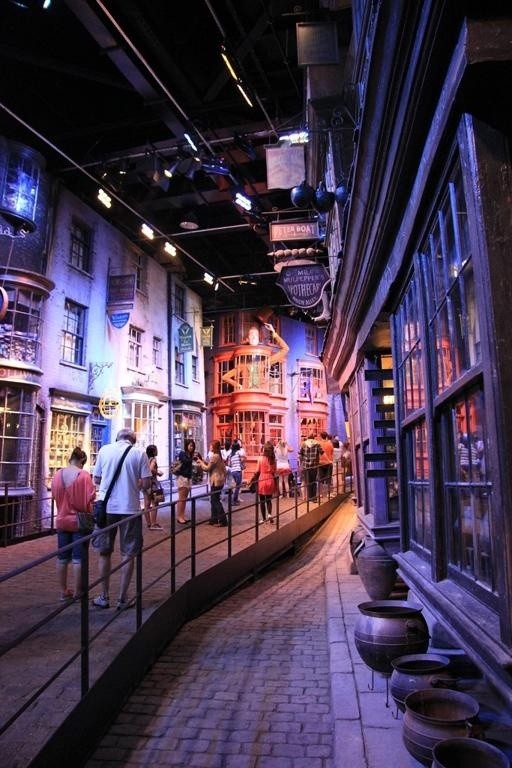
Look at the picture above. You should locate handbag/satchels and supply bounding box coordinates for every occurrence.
[152,489,164,503]
[78,512,95,534]
[170,460,184,475]
[239,454,246,470]
[94,500,107,528]
[246,472,260,493]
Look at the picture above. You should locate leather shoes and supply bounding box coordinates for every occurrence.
[177,519,187,524]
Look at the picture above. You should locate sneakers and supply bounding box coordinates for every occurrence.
[92,596,110,608]
[116,599,135,610]
[59,589,75,601]
[268,514,274,523]
[214,523,223,527]
[150,523,164,530]
[258,519,264,524]
[73,591,81,603]
[145,524,150,529]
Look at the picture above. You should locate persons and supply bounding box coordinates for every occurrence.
[196,429,352,528]
[144,445,164,530]
[454,429,488,484]
[50,446,96,601]
[222,321,290,390]
[173,439,196,524]
[93,427,152,611]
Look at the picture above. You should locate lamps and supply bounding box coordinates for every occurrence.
[188,425,193,436]
[96,129,309,292]
